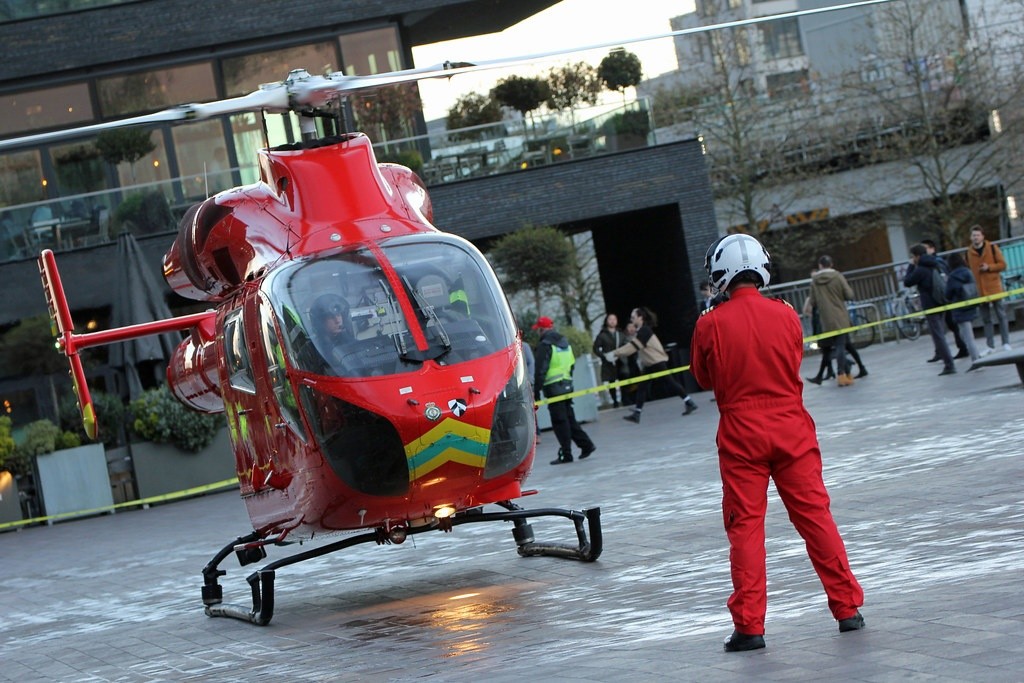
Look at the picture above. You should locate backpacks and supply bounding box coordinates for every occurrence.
[931,265,948,304]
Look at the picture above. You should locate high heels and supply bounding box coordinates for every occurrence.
[823,371,836,380]
[845,359,856,376]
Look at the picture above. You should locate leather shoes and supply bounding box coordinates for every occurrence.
[838,611,866,633]
[724,632,765,652]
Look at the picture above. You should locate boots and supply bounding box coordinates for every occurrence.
[682,396,697,415]
[623,408,641,423]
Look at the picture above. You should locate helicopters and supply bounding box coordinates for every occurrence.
[0,0,889,625]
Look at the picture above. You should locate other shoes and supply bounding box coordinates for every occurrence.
[980,348,997,357]
[1002,343,1012,351]
[550,453,573,465]
[855,371,867,378]
[838,374,854,386]
[579,443,596,459]
[806,376,821,385]
[967,364,983,373]
[927,354,942,363]
[952,350,969,359]
[536,435,541,443]
[938,370,955,376]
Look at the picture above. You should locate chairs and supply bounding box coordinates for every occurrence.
[1,206,111,258]
[107,457,134,500]
[415,275,467,325]
[424,123,597,187]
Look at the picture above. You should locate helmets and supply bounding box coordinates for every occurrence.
[309,294,346,325]
[705,234,771,294]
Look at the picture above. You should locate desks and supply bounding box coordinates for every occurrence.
[438,150,488,182]
[522,132,574,166]
[25,219,91,251]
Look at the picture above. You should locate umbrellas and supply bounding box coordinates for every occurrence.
[107,226,183,400]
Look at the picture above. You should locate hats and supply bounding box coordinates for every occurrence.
[532,317,553,329]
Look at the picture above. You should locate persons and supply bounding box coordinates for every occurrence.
[522,317,596,464]
[905,245,958,376]
[689,234,866,651]
[699,283,721,309]
[612,306,699,424]
[593,312,638,408]
[298,294,355,373]
[803,255,870,385]
[945,252,984,372]
[921,240,968,362]
[962,223,1012,357]
